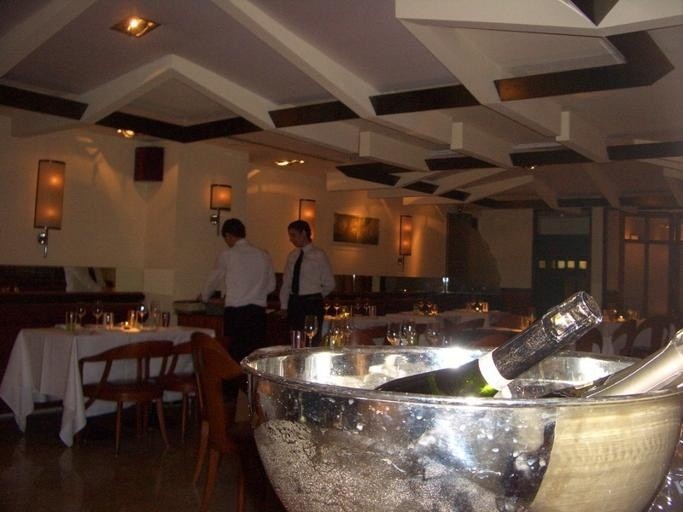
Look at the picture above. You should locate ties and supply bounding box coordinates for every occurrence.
[292,249,304,293]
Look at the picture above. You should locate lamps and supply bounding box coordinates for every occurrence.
[210,183,233,236]
[399,214,413,272]
[299,199,316,240]
[33,158,67,258]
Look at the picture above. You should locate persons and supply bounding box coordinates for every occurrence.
[197,217,275,433]
[278,220,335,348]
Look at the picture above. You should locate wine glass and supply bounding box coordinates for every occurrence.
[333,301,342,318]
[76,304,87,331]
[399,317,419,345]
[322,302,331,319]
[304,314,319,344]
[90,299,104,329]
[137,302,147,330]
[386,317,403,344]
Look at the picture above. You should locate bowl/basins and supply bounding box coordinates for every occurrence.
[240,344,679,511]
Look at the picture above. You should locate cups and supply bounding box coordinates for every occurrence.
[161,311,171,329]
[103,311,114,330]
[291,329,307,349]
[127,309,137,328]
[367,305,377,320]
[65,310,78,334]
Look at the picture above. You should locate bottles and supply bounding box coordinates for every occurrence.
[375,290,602,398]
[533,330,683,398]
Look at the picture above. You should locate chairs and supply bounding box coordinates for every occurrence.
[576,328,602,353]
[620,317,669,359]
[190,331,243,512]
[144,341,202,444]
[611,319,636,357]
[78,340,175,459]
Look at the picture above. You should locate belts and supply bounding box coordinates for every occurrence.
[290,296,324,301]
[225,307,266,312]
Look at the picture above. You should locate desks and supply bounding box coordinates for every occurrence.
[1,324,215,449]
[386,312,445,330]
[315,315,388,338]
[440,311,489,329]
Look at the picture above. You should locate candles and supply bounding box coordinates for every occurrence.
[617,315,624,322]
[124,321,130,329]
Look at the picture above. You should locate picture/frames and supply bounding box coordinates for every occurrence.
[331,210,381,248]
